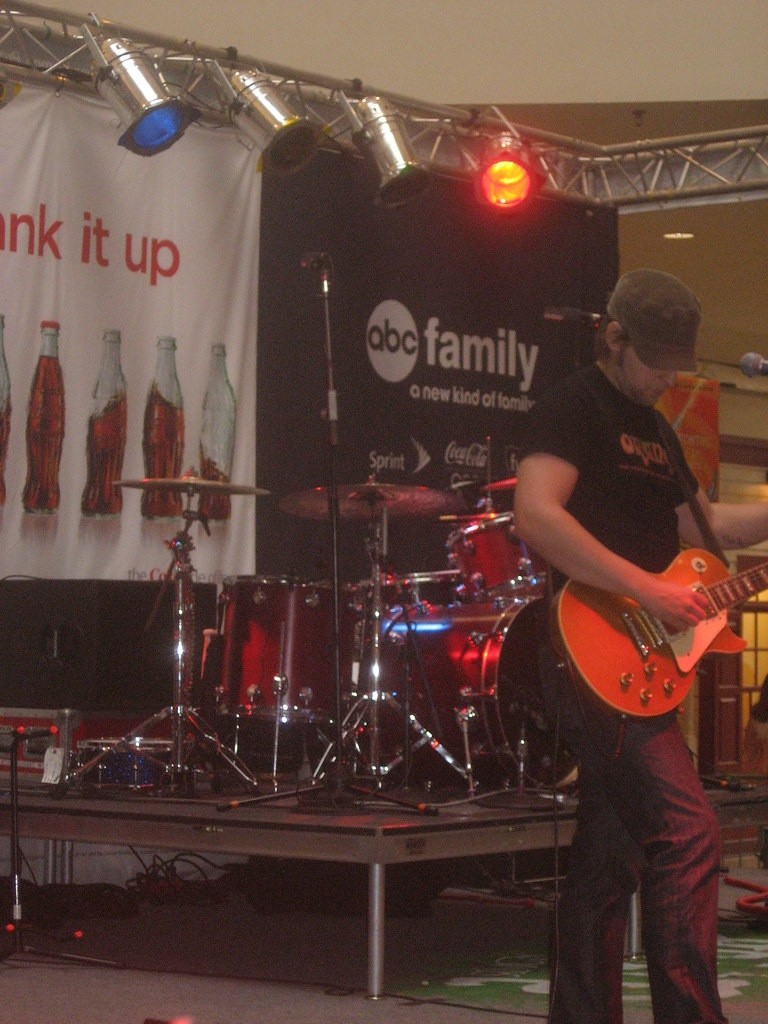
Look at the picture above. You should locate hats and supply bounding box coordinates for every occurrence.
[607,268,702,374]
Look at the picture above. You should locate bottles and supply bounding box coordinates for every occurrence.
[198,345,235,517]
[141,336,185,518]
[22,319,66,515]
[80,330,127,519]
[0,315,12,510]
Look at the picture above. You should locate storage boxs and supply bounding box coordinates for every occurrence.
[0,706,174,785]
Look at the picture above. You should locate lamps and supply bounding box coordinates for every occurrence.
[458,103,545,216]
[81,23,199,161]
[339,89,437,212]
[208,58,329,179]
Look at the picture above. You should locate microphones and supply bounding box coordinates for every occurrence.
[300,250,329,276]
[740,352,768,377]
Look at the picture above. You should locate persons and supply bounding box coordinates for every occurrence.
[512,269,768,1024]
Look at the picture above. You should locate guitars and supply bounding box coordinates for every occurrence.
[547,547,768,725]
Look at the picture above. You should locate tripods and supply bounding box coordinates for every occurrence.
[0,727,135,976]
[49,266,491,817]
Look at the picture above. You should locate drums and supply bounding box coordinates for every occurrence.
[446,510,550,606]
[361,568,465,604]
[376,601,584,794]
[211,572,356,736]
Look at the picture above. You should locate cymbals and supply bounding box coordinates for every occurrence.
[277,481,467,521]
[112,477,270,496]
[479,477,518,494]
